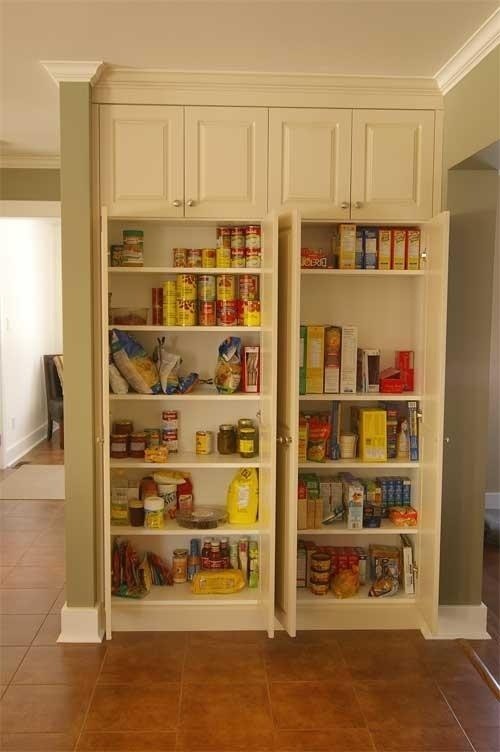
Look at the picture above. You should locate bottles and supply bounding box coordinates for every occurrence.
[202,537,211,569]
[220,536,230,568]
[111,471,129,524]
[208,540,221,569]
[387,408,396,457]
[240,537,249,582]
[249,541,259,588]
[230,543,239,570]
[188,539,201,582]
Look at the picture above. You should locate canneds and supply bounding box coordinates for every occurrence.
[196,430,213,455]
[231,226,246,249]
[146,428,161,448]
[152,306,163,325]
[309,578,330,594]
[217,249,230,267]
[176,273,197,299]
[163,304,176,326]
[230,249,245,267]
[111,434,128,457]
[188,248,201,267]
[157,484,178,519]
[144,496,165,530]
[172,248,187,268]
[246,249,261,268]
[217,275,238,301]
[311,553,330,572]
[162,411,179,431]
[239,275,259,300]
[217,226,230,248]
[162,431,179,453]
[310,567,329,583]
[238,300,260,325]
[113,420,132,435]
[217,425,236,454]
[198,301,216,325]
[176,300,198,325]
[241,428,256,457]
[123,231,144,267]
[152,287,163,306]
[246,225,261,248]
[163,280,176,304]
[172,549,187,583]
[129,499,145,527]
[217,301,237,325]
[130,431,147,457]
[202,248,217,268]
[236,419,253,453]
[198,274,216,300]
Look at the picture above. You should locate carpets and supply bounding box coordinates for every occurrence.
[1,464,66,500]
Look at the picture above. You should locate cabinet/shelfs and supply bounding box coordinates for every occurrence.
[90,211,280,638]
[96,104,265,218]
[277,214,447,642]
[268,105,433,222]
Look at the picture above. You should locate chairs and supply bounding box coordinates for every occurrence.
[38,352,65,449]
